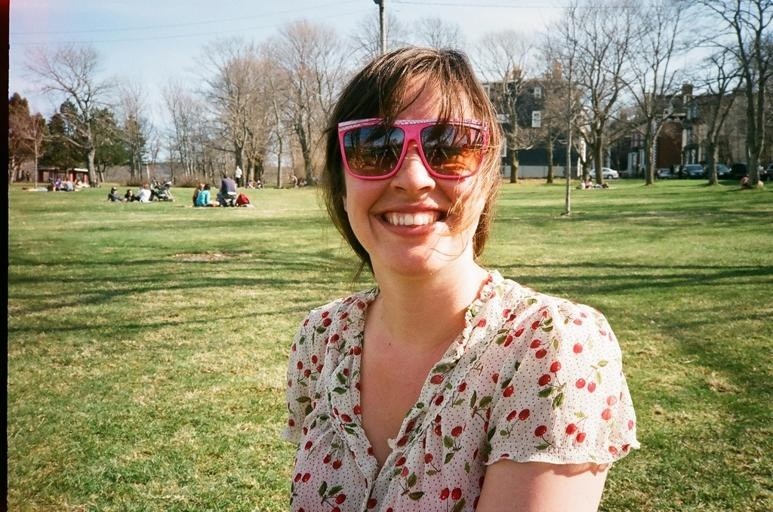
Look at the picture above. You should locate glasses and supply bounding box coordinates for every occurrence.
[338,119,490,180]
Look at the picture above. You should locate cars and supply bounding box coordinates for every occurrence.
[591,167,620,179]
[656,162,773,181]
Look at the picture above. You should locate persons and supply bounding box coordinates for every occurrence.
[234,164,299,189]
[47,174,90,192]
[277,47,642,512]
[107,178,173,202]
[740,173,764,189]
[192,175,250,207]
[576,174,609,189]
